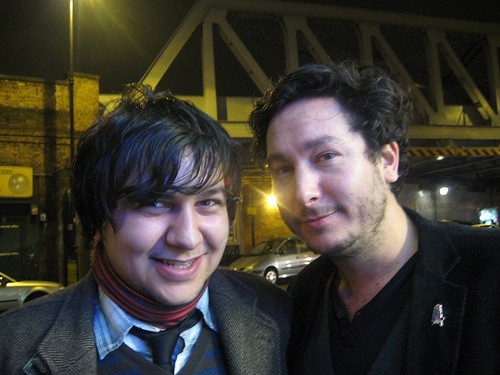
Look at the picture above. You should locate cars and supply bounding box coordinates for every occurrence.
[227,236,324,286]
[1,273,65,311]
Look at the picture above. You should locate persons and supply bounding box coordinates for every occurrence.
[239,67,500,375]
[1,96,289,374]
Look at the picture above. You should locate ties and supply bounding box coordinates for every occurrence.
[128,308,203,375]
